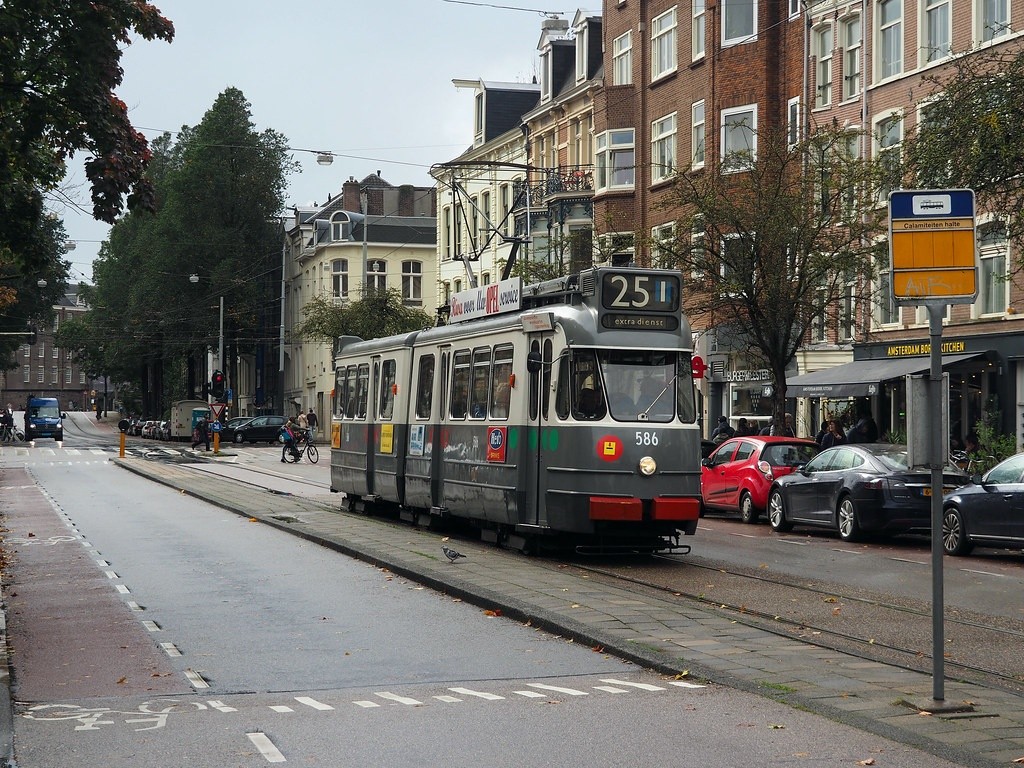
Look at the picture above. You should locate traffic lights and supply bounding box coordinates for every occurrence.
[212,370,225,398]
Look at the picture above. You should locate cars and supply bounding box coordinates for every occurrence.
[767,443,970,542]
[942,452,1024,557]
[700,436,823,524]
[124,420,171,440]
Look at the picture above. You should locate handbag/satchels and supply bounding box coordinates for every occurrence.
[306,426,310,431]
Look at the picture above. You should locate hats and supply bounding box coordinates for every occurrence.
[717,416,726,423]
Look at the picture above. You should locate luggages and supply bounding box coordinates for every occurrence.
[12,427,24,441]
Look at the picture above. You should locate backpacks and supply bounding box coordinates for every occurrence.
[713,432,730,446]
[195,421,203,432]
[277,425,292,444]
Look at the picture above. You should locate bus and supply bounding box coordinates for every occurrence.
[330,266,701,560]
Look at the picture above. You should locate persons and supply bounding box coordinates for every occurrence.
[951,435,988,468]
[96,405,102,420]
[280,410,319,463]
[456,382,513,420]
[0,402,23,443]
[712,413,796,445]
[192,412,212,451]
[815,411,879,453]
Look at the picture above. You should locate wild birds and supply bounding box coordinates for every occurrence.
[440,545,467,563]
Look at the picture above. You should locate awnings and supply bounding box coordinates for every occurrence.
[761,352,996,400]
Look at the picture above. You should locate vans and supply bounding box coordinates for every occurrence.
[24,398,66,440]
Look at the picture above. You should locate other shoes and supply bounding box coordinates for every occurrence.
[191,443,195,450]
[281,459,286,463]
[294,459,300,462]
[206,449,212,451]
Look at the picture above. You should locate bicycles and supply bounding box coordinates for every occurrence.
[0,424,25,443]
[283,429,319,464]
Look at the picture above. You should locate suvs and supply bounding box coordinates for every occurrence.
[212,417,258,444]
[233,416,288,444]
